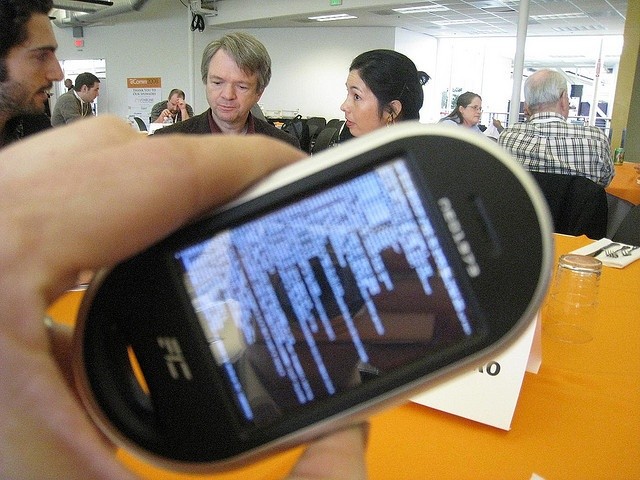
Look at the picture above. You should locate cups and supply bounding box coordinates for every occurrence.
[542,254,602,345]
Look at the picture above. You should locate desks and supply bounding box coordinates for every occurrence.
[44,231,640,480]
[603,159,640,241]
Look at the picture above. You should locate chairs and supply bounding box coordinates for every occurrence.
[134,117,148,131]
[518,172,608,245]
[303,116,350,157]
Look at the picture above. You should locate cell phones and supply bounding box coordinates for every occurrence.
[70,120,554,471]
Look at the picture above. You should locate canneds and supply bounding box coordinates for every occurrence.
[614,147,625,165]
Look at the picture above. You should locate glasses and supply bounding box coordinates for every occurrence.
[467,104,483,111]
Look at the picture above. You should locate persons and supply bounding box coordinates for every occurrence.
[63,78,75,93]
[0,0,64,148]
[435,92,490,142]
[492,99,530,135]
[498,69,615,240]
[0,114,365,480]
[150,89,193,123]
[310,50,423,154]
[149,32,302,148]
[51,72,100,126]
